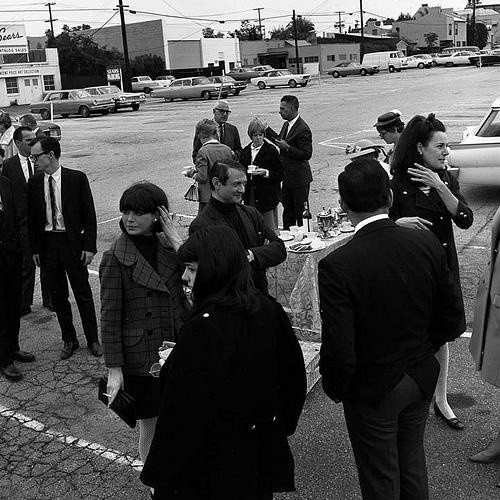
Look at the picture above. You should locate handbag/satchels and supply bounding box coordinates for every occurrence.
[184,183,199,201]
[99,378,138,428]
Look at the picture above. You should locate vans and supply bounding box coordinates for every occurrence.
[362,51,409,73]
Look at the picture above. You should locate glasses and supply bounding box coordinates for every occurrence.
[31,152,45,161]
[216,107,231,114]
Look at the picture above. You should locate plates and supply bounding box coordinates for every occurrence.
[246,170,254,174]
[279,236,293,241]
[182,171,188,175]
[287,241,327,254]
[341,227,355,232]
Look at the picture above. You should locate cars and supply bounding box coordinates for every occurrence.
[405,45,500,69]
[225,64,274,81]
[149,76,234,103]
[156,76,176,82]
[446,96,500,188]
[29,89,115,120]
[1,109,62,143]
[81,86,148,112]
[251,69,312,90]
[202,76,249,96]
[326,61,380,78]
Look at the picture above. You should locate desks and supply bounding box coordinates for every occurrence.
[266,219,355,342]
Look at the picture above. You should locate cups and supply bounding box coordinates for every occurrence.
[289,226,298,231]
[342,222,352,230]
[159,341,178,362]
[280,231,289,238]
[184,165,193,174]
[248,165,258,171]
[305,232,317,241]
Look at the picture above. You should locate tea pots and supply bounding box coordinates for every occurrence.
[317,204,349,240]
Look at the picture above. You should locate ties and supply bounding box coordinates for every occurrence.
[48,175,57,231]
[218,124,223,142]
[26,157,34,179]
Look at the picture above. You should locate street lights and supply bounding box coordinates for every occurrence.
[468,0,482,46]
[120,9,136,89]
[360,10,366,63]
[291,9,302,74]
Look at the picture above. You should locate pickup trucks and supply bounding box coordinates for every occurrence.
[131,76,170,94]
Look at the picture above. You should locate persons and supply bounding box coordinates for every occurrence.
[185,119,234,212]
[192,100,244,165]
[141,225,307,500]
[189,159,287,293]
[469,206,500,462]
[382,114,474,431]
[99,183,193,494]
[374,111,405,163]
[318,156,455,499]
[239,121,283,229]
[252,95,312,228]
[0,113,103,381]
[347,138,394,182]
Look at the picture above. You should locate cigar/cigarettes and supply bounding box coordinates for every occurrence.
[102,393,110,397]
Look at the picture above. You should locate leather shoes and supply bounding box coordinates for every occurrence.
[61,338,79,359]
[434,401,464,429]
[468,438,499,462]
[2,363,22,380]
[89,341,103,356]
[14,350,35,362]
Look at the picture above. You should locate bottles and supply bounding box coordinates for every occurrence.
[302,202,313,237]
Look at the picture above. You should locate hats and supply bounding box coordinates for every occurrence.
[373,113,399,127]
[345,139,383,159]
